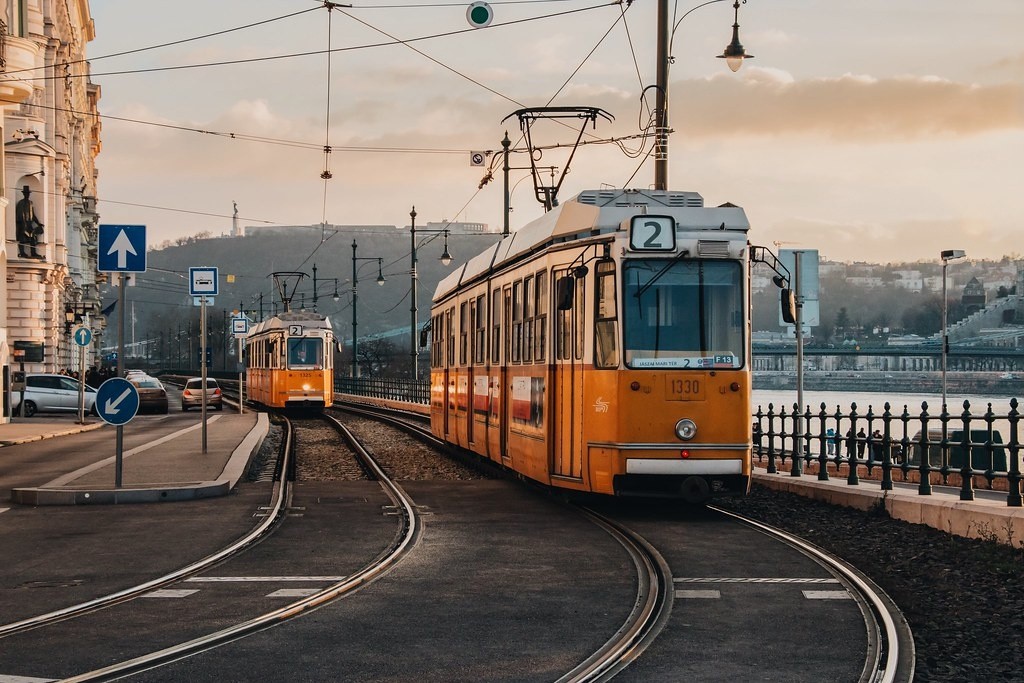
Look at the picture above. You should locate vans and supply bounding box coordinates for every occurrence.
[908,428,1007,473]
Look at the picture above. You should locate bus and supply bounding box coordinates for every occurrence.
[419,190,796,504]
[245,313,343,410]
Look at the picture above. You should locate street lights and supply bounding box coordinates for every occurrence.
[409,206,455,381]
[940,249,967,405]
[312,263,340,313]
[654,0,755,191]
[350,238,389,376]
[501,130,560,238]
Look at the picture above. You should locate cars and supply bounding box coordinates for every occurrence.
[9,374,100,417]
[178,378,223,412]
[123,369,169,416]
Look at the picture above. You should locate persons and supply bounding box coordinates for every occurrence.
[298,350,309,363]
[827,428,834,455]
[834,430,842,455]
[874,430,882,438]
[857,428,866,459]
[16,186,44,258]
[59,366,118,380]
[845,428,851,457]
